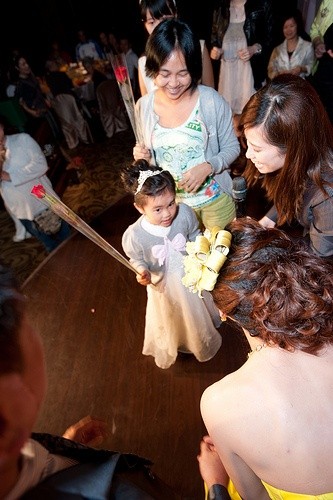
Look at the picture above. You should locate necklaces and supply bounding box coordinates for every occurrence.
[247,343,266,357]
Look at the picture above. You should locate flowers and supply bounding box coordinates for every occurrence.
[182,226,232,298]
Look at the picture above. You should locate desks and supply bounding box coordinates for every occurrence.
[46,61,114,106]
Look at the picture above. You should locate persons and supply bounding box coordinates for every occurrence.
[1,31,139,159]
[135,0,333,145]
[0,124,71,253]
[237,72,333,257]
[0,261,171,500]
[121,160,222,369]
[182,215,333,500]
[132,17,242,234]
[196,436,233,500]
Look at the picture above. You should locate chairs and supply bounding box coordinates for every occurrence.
[96,78,128,137]
[52,94,93,150]
